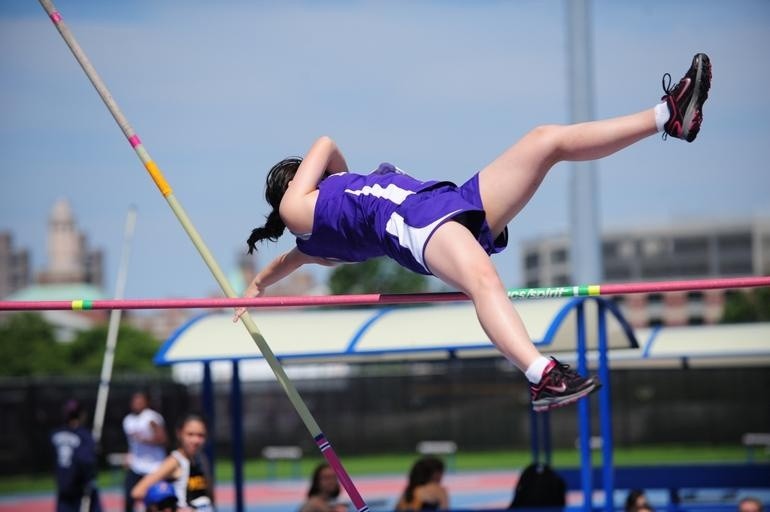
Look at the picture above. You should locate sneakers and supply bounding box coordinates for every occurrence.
[528,353,604,414]
[661,49,714,144]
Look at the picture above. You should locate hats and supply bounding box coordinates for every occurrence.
[143,477,180,506]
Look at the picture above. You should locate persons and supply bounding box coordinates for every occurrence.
[129,415,216,512]
[392,457,449,511]
[52,397,103,512]
[122,387,169,512]
[627,490,656,511]
[299,462,352,511]
[232,51,713,414]
[145,479,178,512]
[739,495,762,511]
[508,462,569,511]
